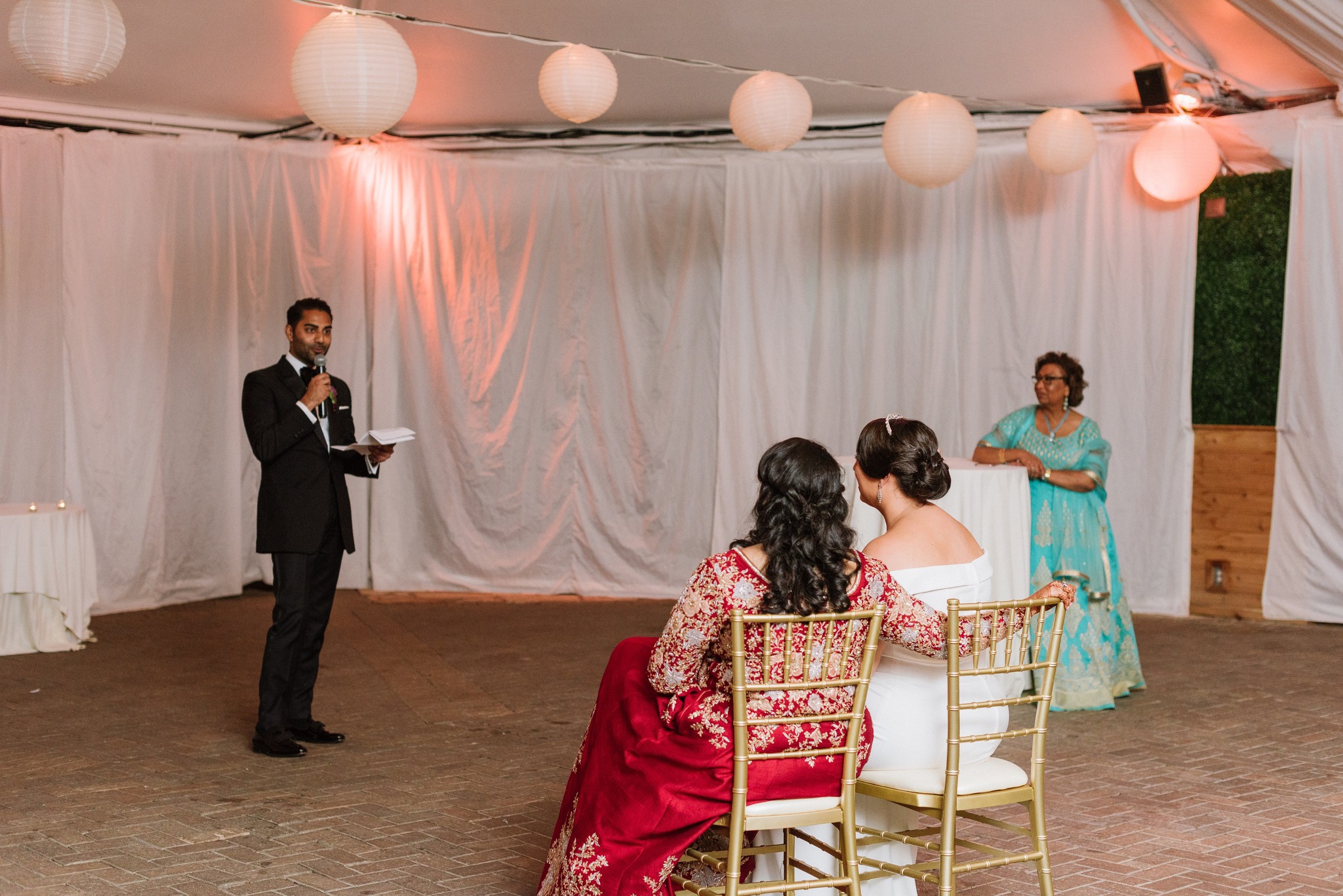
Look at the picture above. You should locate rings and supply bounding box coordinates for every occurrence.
[1061,581,1068,590]
[1030,467,1035,471]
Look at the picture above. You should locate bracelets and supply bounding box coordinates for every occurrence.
[998,448,1006,466]
[1042,467,1052,483]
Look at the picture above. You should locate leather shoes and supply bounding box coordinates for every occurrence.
[293,719,345,745]
[252,736,308,758]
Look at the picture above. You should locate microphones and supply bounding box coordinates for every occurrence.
[314,354,328,419]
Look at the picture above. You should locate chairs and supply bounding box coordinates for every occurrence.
[833,598,1066,896]
[669,604,886,896]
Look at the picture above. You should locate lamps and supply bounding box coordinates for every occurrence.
[1174,88,1202,113]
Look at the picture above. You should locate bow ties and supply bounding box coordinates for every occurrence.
[299,366,326,387]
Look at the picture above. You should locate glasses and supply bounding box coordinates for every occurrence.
[1031,375,1066,385]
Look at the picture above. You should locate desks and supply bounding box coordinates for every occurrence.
[823,452,1032,689]
[0,502,99,656]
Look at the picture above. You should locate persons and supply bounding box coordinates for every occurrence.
[534,437,1077,896]
[972,352,1145,714]
[751,414,1010,896]
[242,297,394,760]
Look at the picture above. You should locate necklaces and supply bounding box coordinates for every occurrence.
[1040,406,1070,441]
